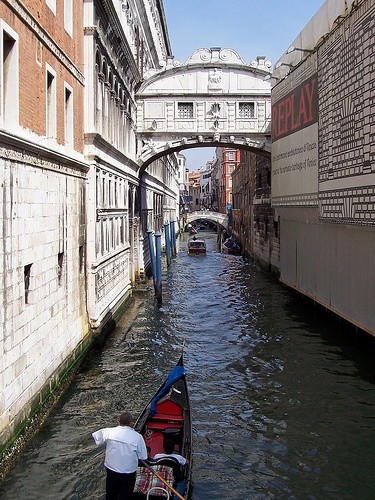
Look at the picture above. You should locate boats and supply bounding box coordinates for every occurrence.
[199,219,215,230]
[221,235,241,254]
[190,226,199,235]
[187,236,206,258]
[133,339,193,499]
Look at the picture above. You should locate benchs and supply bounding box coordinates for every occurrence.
[138,455,181,485]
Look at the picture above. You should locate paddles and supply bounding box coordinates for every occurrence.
[142,461,186,500]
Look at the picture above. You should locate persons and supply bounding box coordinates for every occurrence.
[144,447,157,462]
[92,412,148,500]
[153,436,190,466]
[200,206,206,215]
[226,203,232,215]
